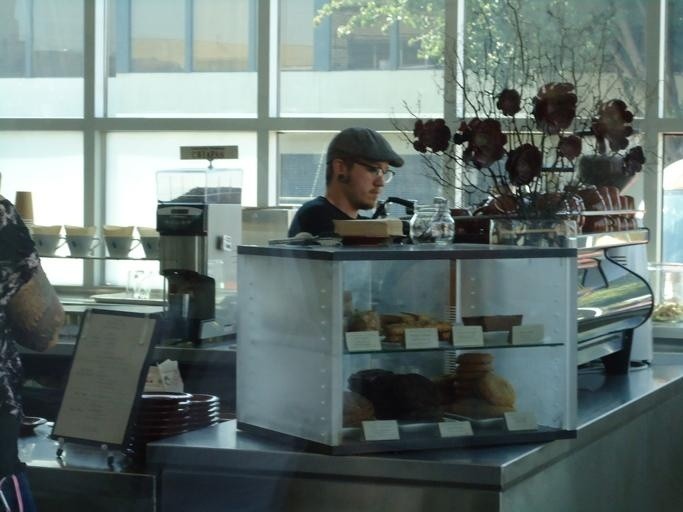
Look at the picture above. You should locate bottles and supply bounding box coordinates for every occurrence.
[410,203,456,246]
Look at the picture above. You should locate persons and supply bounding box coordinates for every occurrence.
[0,195,66,512]
[286,123,406,238]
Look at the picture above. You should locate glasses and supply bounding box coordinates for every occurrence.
[342,156,396,186]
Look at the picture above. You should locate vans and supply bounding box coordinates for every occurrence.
[631,158,683,341]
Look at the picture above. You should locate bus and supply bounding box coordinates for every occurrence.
[154,128,647,328]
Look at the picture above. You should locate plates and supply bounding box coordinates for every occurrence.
[126,390,220,472]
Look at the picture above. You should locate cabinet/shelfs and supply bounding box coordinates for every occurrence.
[234,243,578,455]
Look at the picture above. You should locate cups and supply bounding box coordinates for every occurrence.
[31,233,161,260]
[126,269,151,299]
[15,191,33,230]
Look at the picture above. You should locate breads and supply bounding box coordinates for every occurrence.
[343,311,517,425]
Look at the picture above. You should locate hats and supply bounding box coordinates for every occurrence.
[327,126,404,167]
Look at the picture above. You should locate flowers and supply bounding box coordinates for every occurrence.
[389,0,660,218]
